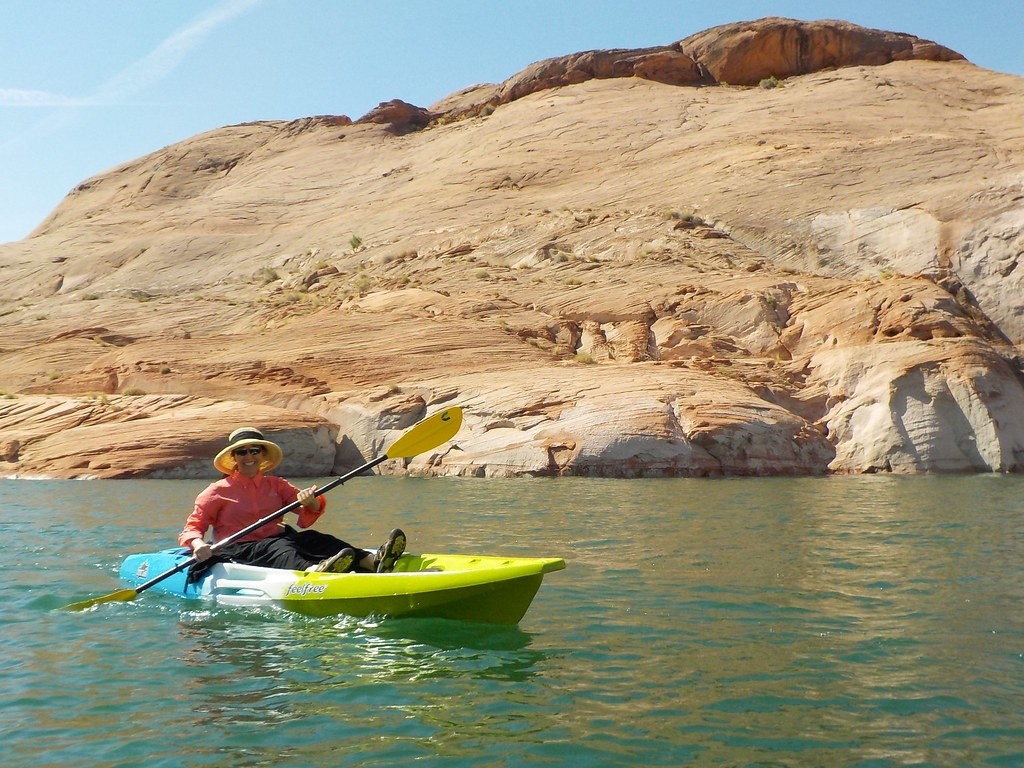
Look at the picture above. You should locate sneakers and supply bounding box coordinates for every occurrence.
[371,527,406,574]
[312,546,356,573]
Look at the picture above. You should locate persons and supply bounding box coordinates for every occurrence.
[177,427,407,572]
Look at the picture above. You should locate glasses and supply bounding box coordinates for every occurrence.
[232,447,262,456]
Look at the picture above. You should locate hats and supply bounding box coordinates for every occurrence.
[213,427,283,476]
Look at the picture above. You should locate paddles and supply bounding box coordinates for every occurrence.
[53,405,463,612]
